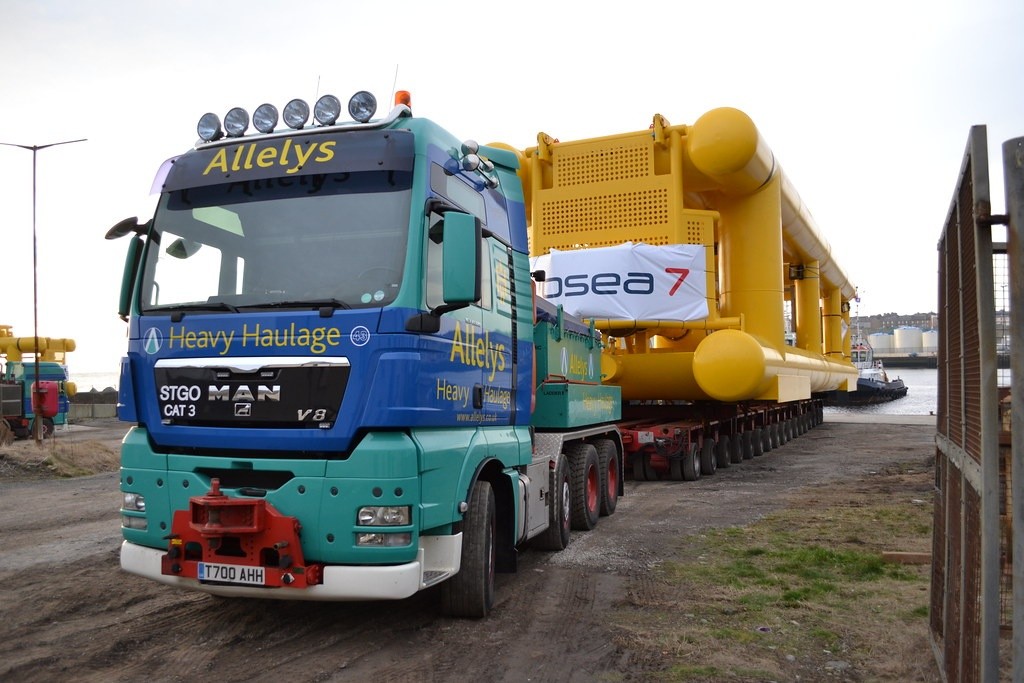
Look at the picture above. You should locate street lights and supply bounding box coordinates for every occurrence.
[0,135,87,444]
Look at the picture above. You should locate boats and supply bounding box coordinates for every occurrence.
[817,285,909,405]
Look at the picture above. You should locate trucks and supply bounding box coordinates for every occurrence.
[0,361,77,449]
[103,88,628,621]
